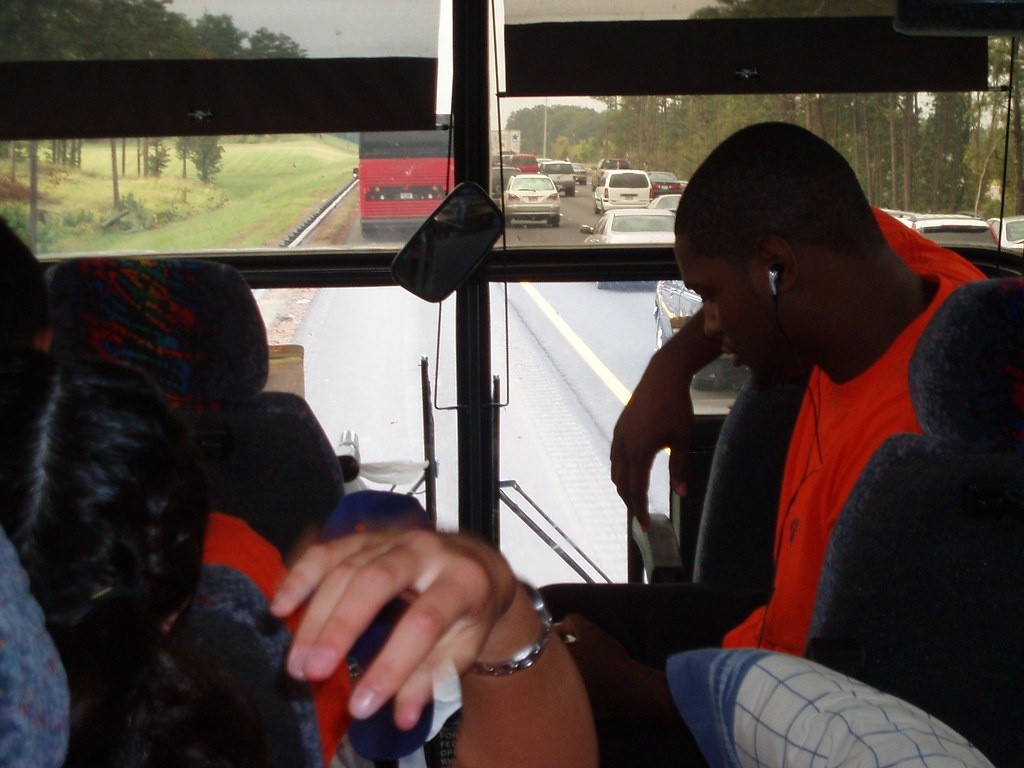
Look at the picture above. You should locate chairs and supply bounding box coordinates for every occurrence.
[43,257,342,555]
[538,245,1024,768]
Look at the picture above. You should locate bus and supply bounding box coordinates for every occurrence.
[352,114,454,239]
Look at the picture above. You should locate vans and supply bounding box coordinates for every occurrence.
[592,169,653,217]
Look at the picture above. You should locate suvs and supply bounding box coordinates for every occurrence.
[499,173,561,227]
[880,208,999,246]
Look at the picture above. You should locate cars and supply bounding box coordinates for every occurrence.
[538,161,576,197]
[571,163,587,185]
[647,194,682,215]
[506,154,539,173]
[492,167,522,208]
[493,151,517,167]
[986,216,1024,250]
[590,158,632,192]
[579,208,676,245]
[647,172,682,199]
[653,279,750,390]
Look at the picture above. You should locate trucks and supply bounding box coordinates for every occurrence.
[490,128,520,157]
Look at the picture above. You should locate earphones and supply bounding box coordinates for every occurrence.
[768,263,783,297]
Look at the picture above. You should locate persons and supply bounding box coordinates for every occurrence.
[553,122,991,717]
[1,217,599,767]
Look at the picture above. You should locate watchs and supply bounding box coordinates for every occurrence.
[472,582,552,676]
